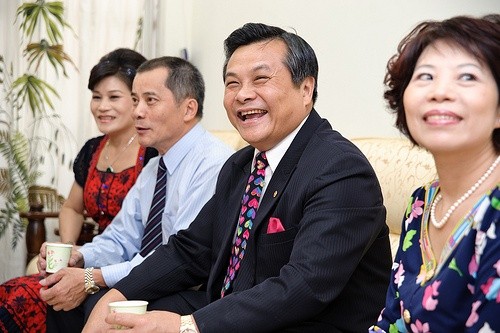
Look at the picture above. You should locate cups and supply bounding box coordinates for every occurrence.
[108,300,148,329]
[45,243,73,274]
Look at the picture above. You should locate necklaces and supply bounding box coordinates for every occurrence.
[102,131,137,174]
[430,155,500,230]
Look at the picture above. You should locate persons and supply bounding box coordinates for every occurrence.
[367,13,500,333]
[0,46,160,333]
[82,22,393,333]
[37,56,237,333]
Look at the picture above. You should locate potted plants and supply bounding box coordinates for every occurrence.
[0,0,82,268]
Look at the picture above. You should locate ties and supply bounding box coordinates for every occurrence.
[134,158,167,257]
[221,153,269,298]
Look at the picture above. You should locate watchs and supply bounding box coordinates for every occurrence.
[180,315,198,333]
[84,267,100,294]
